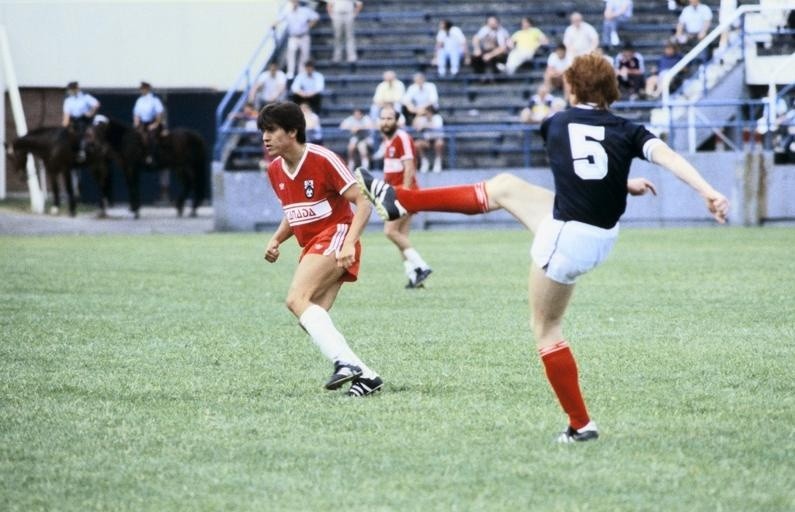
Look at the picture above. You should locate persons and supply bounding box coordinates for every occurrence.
[339,70,447,173]
[132,83,169,171]
[671,0,714,47]
[375,103,434,293]
[602,0,632,47]
[644,44,682,98]
[431,15,645,124]
[58,79,103,143]
[352,51,733,446]
[241,0,366,145]
[256,99,385,399]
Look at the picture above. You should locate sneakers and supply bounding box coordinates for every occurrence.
[345,377,384,398]
[404,267,432,288]
[555,420,599,446]
[355,167,409,220]
[325,361,363,389]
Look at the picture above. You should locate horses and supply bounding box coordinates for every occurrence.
[4,126,123,215]
[69,112,209,219]
